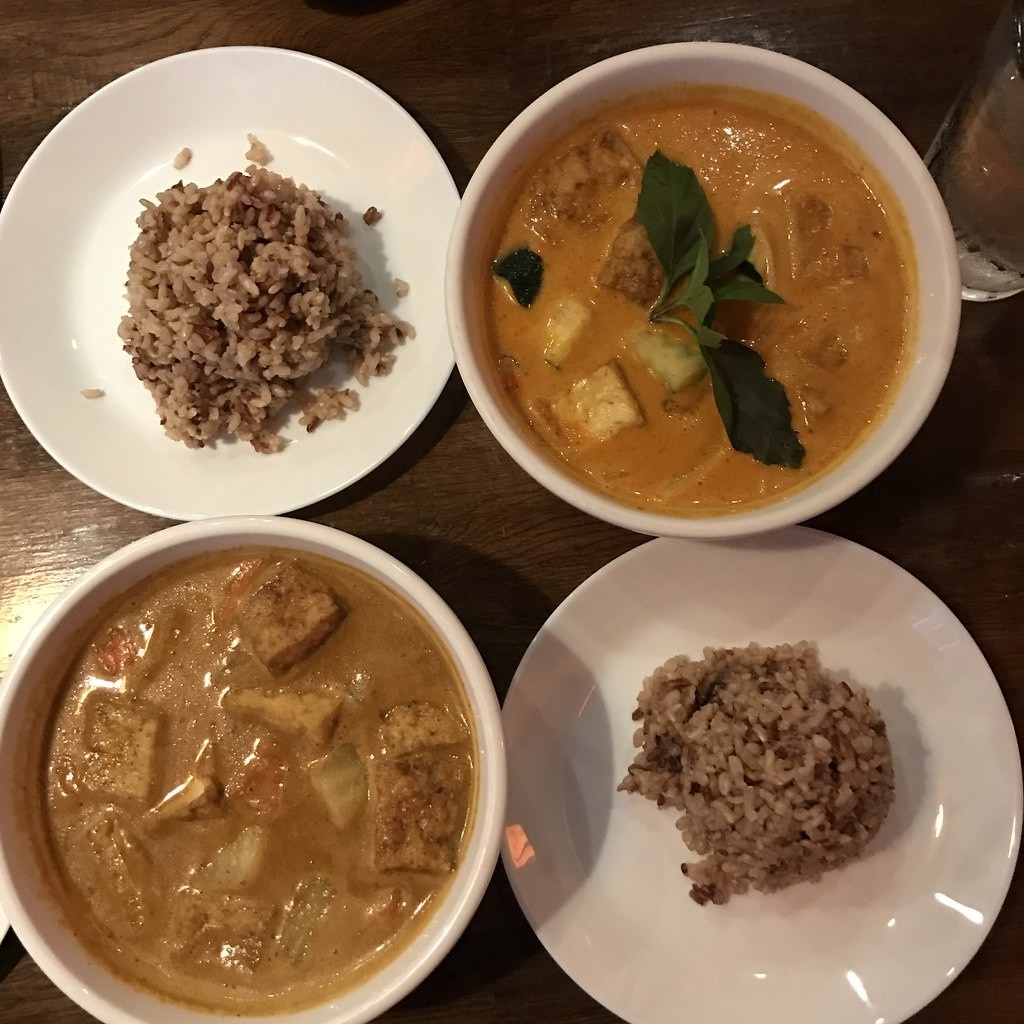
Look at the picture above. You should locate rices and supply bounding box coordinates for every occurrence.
[117,131,416,453]
[617,641,896,905]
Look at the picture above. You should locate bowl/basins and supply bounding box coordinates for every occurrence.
[0,516,507,1024]
[443,39,959,538]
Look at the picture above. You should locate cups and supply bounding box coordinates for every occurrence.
[924,0,1024,302]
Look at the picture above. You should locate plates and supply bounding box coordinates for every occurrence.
[502,529,1024,1024]
[0,45,454,520]
